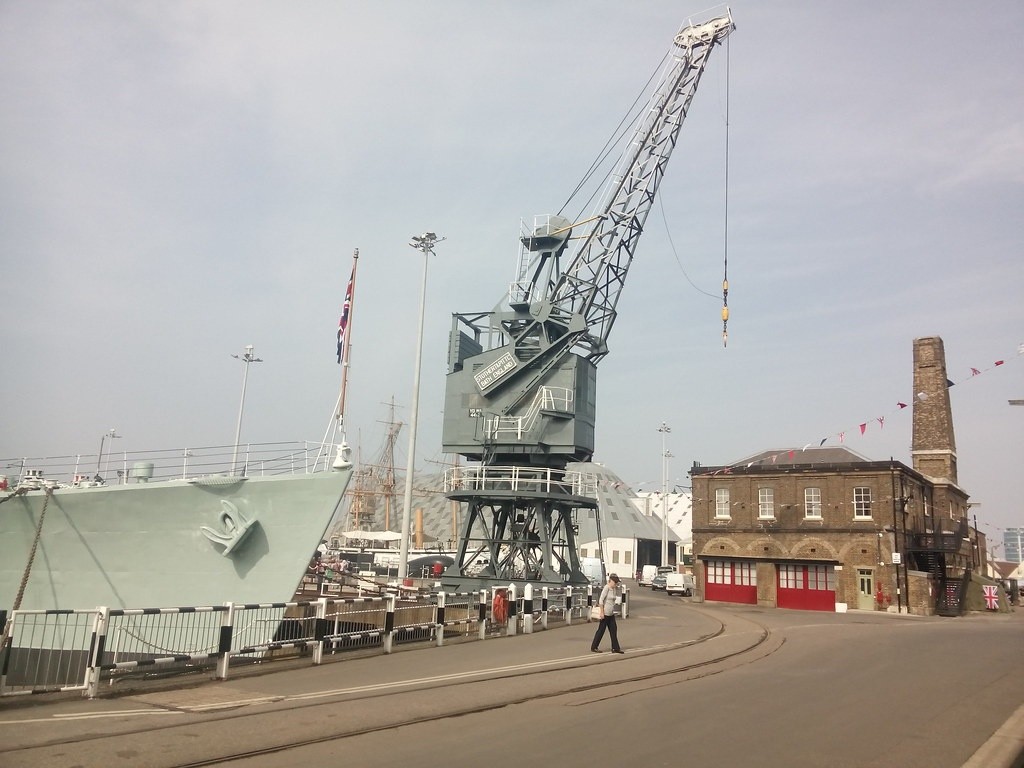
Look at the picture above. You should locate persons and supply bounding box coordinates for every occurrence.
[1018,586,1024,607]
[308,561,333,590]
[590,574,625,654]
[324,558,353,573]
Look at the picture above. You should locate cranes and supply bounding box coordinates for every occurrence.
[372,5,738,590]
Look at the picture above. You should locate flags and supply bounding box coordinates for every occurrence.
[982,584,999,609]
[947,583,959,605]
[337,267,355,364]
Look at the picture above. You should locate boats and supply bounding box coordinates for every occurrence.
[0,248,361,689]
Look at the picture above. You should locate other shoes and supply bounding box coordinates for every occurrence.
[612,649,624,653]
[591,647,602,653]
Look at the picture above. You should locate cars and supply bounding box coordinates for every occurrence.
[652,576,666,591]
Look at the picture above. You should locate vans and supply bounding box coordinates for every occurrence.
[666,574,693,596]
[638,564,658,586]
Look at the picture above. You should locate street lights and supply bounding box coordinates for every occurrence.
[229,344,262,477]
[104,428,121,481]
[397,231,441,585]
[659,423,672,568]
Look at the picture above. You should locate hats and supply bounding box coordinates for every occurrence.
[610,573,621,582]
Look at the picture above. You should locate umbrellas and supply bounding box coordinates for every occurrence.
[342,529,401,541]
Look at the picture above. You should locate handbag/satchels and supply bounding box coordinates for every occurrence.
[591,604,604,620]
